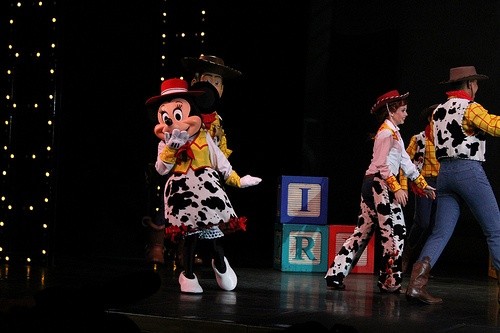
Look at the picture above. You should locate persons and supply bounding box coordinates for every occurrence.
[145,78,264,293]
[399,104,440,275]
[148,55,244,274]
[404,65,500,306]
[324,90,438,294]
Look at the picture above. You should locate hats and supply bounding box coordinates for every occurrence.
[440,65,488,84]
[419,103,439,125]
[371,88,409,113]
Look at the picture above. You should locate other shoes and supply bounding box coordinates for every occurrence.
[327,280,346,288]
[380,287,406,294]
[402,260,408,276]
[429,272,436,278]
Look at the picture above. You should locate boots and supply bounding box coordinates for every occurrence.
[496,271,500,304]
[406,257,446,304]
